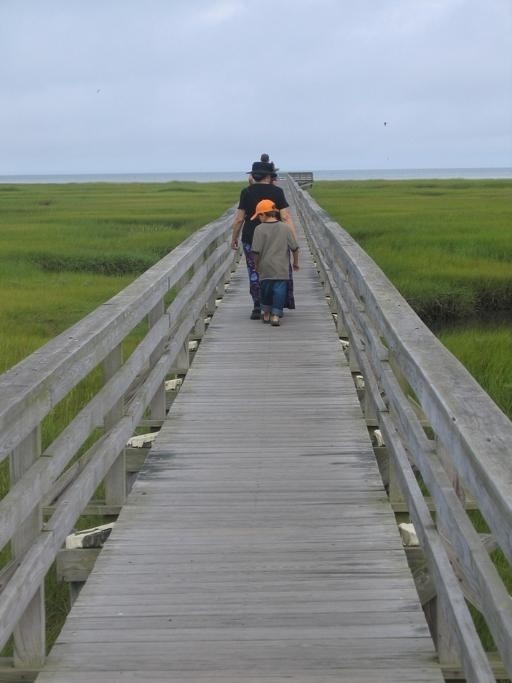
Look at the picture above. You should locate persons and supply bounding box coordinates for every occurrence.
[230,152,299,327]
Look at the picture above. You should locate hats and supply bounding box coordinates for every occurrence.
[251,200,275,221]
[247,162,277,177]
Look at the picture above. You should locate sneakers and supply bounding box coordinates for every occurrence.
[251,309,261,319]
[263,318,280,325]
